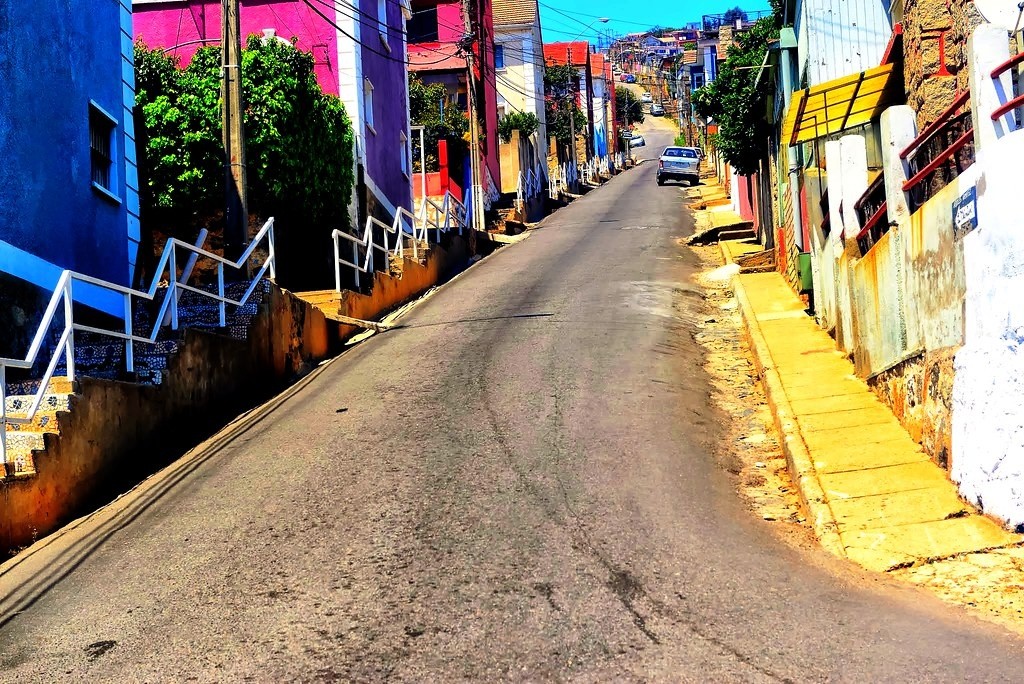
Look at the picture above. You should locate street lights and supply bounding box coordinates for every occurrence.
[660,69,681,137]
[566,18,609,195]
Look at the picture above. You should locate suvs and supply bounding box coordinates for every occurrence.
[656,146,700,186]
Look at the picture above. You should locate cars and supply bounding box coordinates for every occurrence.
[681,147,704,160]
[642,92,653,103]
[649,104,665,117]
[623,132,632,137]
[630,135,646,148]
[612,64,636,83]
